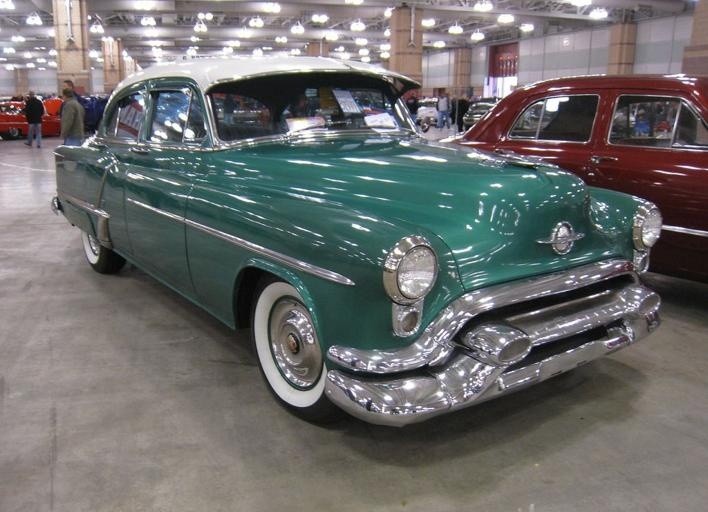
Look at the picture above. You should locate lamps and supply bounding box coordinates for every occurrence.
[306,1,392,63]
[421,15,435,29]
[447,22,463,35]
[468,29,486,42]
[567,1,611,22]
[473,2,535,33]
[433,40,446,49]
[0,1,305,71]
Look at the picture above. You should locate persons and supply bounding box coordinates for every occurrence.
[9,78,696,147]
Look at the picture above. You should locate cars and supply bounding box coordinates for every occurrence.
[50,56,673,436]
[433,70,707,283]
